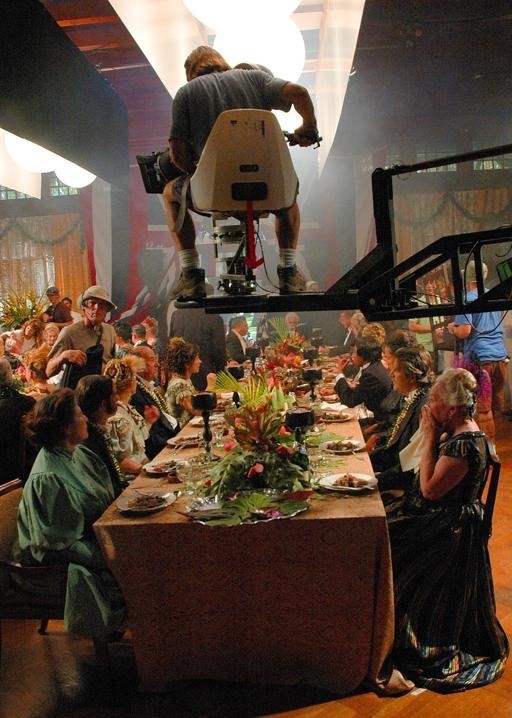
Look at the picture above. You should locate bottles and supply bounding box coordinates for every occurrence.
[290,427,311,485]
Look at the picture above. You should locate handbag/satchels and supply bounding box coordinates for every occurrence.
[60,344,104,390]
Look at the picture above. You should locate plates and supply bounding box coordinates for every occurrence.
[317,473,380,493]
[320,440,365,453]
[166,434,208,447]
[145,459,190,476]
[189,415,224,426]
[320,412,354,422]
[114,489,176,511]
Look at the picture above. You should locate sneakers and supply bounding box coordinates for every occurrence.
[276,263,307,294]
[168,268,207,301]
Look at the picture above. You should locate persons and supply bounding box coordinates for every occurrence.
[0,283,299,431]
[160,45,320,302]
[16,340,248,695]
[446,258,512,464]
[324,283,508,695]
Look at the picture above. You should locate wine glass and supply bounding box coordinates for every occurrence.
[175,463,206,509]
[305,439,335,492]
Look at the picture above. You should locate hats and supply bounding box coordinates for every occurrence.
[45,287,57,294]
[461,261,488,282]
[83,286,116,312]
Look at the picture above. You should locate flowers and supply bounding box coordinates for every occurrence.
[203,315,322,502]
[1,274,53,332]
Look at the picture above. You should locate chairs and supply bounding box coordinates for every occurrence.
[189,108,300,295]
[0,478,113,691]
[477,438,502,542]
